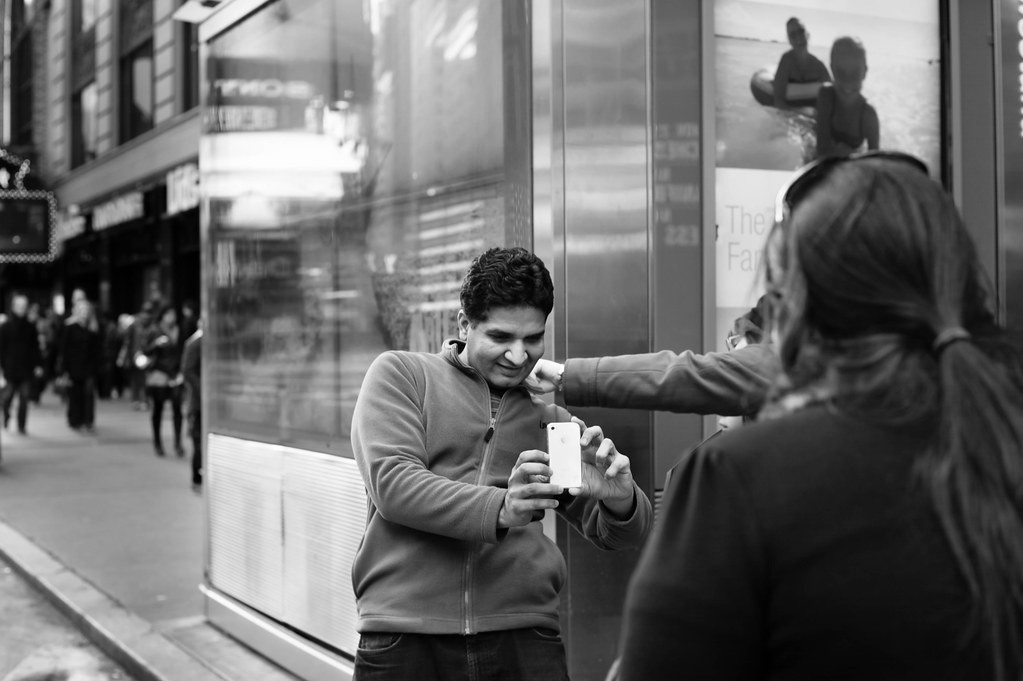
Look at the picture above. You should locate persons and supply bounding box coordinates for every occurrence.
[613,151,1023,681]
[1,286,203,485]
[816,35,880,156]
[351,249,652,681]
[526,299,783,424]
[774,16,830,107]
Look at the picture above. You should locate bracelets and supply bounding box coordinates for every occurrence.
[557,364,564,391]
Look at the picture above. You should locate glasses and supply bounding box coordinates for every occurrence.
[775,151,929,272]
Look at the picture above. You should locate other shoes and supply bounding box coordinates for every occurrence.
[175,444,184,456]
[192,472,201,483]
[156,446,163,454]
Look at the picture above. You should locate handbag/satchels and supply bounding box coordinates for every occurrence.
[133,351,155,372]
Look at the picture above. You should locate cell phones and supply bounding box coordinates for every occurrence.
[547,421,583,489]
